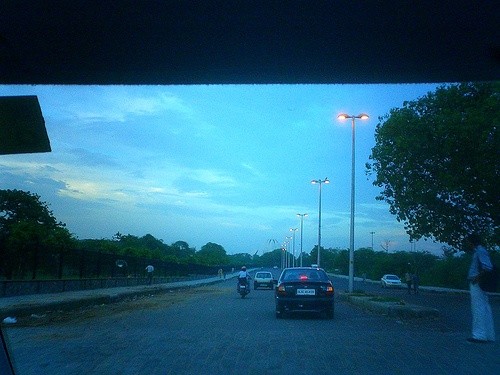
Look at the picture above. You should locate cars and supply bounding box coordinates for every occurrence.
[272,268,334,320]
[381,275,403,290]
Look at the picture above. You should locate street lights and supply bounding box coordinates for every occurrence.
[289,227,299,268]
[283,241,290,268]
[281,244,286,268]
[297,213,308,268]
[337,112,370,293]
[311,177,330,268]
[285,236,293,267]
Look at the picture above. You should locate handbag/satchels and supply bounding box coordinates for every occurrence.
[477,252,497,292]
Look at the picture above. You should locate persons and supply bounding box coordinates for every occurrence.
[405,270,413,294]
[410,272,420,294]
[467,235,497,342]
[238,266,252,294]
[145,263,154,285]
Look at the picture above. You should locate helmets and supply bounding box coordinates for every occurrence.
[241,266,246,270]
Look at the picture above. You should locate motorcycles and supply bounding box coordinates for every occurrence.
[236,276,251,298]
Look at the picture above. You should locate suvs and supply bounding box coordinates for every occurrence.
[253,271,274,290]
[273,265,278,270]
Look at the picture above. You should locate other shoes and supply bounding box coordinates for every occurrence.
[485,340,495,345]
[461,338,487,346]
